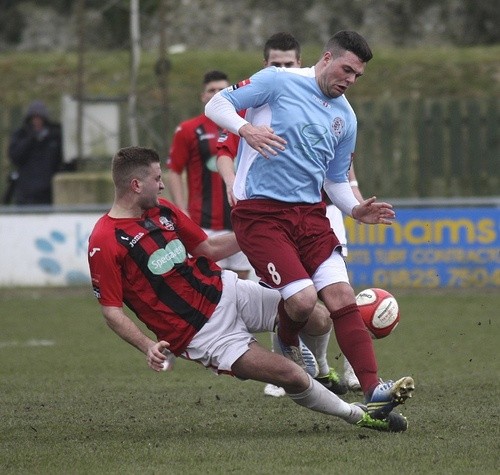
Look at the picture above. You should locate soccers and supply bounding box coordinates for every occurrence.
[355,287,399,339]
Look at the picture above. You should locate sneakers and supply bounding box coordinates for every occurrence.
[276,326,320,379]
[366,375,415,419]
[345,368,360,390]
[315,366,346,394]
[349,402,409,431]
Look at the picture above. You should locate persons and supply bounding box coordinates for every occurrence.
[205,31,415,419]
[88,146,409,433]
[166,31,363,397]
[6,103,60,207]
